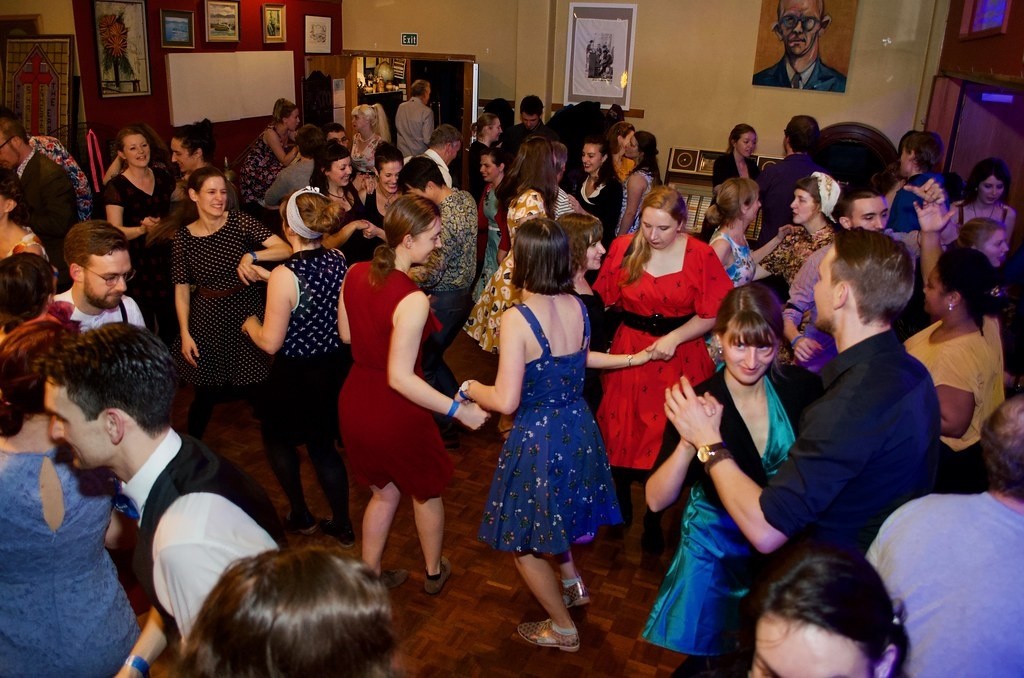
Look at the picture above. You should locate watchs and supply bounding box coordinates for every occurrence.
[628,354,633,367]
[698,440,726,463]
[459,380,476,402]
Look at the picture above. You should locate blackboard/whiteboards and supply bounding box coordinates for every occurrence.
[301,69,334,128]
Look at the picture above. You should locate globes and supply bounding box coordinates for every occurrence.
[374,61,394,83]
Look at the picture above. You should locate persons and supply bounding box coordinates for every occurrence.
[0,80,1024,678]
[586,36,613,79]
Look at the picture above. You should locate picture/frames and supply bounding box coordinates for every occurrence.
[203,0,240,42]
[91,0,153,99]
[0,34,74,156]
[563,2,638,111]
[263,4,287,43]
[159,8,196,50]
[304,14,332,55]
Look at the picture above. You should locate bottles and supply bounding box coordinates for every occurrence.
[367,73,373,87]
[377,79,384,93]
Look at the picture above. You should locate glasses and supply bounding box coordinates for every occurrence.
[79,265,136,286]
[334,137,350,144]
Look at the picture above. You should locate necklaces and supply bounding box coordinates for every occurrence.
[973,203,995,219]
[377,183,393,210]
[358,131,375,143]
[328,189,347,202]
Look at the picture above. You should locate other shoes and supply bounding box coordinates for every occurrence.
[606,513,632,543]
[380,569,408,590]
[315,517,355,545]
[639,546,664,572]
[287,512,317,534]
[424,556,451,594]
[441,436,462,449]
[518,618,580,652]
[561,581,591,608]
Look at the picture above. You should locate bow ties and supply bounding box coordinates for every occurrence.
[113,478,140,520]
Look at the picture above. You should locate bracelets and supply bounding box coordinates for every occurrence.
[125,656,149,677]
[291,150,296,157]
[791,336,803,349]
[459,389,469,401]
[52,271,58,277]
[249,251,257,263]
[705,451,733,475]
[448,402,461,418]
